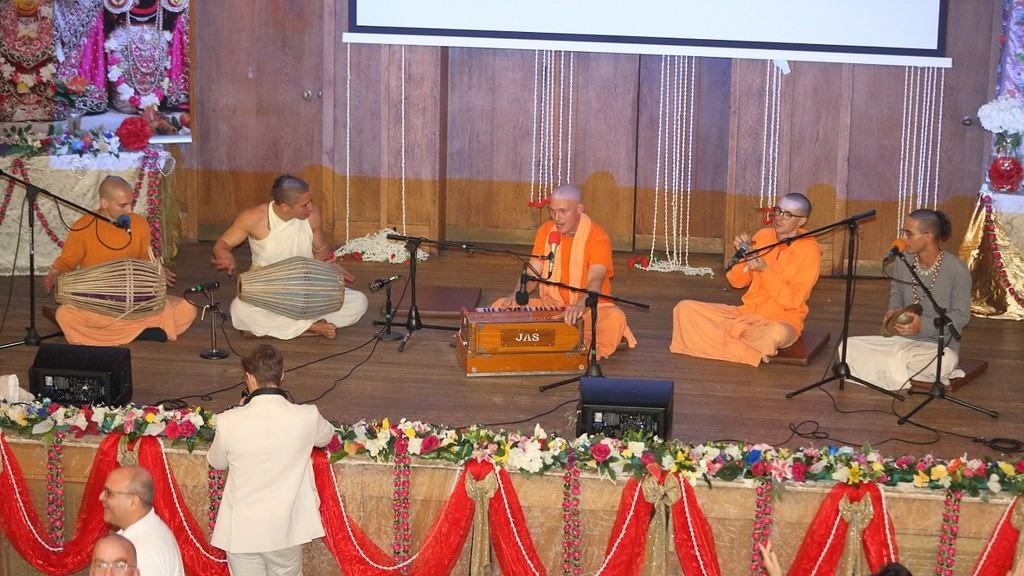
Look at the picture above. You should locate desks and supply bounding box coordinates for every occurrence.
[0,147,183,277]
[956,184,1024,322]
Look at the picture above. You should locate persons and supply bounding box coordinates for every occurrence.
[44,176,197,347]
[207,343,334,576]
[669,193,822,368]
[838,209,972,390]
[491,184,638,361]
[209,175,369,340]
[90,533,140,576]
[99,465,186,576]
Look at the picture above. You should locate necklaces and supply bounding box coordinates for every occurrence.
[911,245,943,305]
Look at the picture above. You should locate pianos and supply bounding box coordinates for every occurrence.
[455,302,588,375]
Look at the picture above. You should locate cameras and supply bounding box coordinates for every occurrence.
[242,388,250,398]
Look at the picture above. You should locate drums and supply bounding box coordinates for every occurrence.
[236,255,346,321]
[55,245,166,320]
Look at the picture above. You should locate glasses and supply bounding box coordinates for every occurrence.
[241,375,246,383]
[773,206,808,221]
[899,229,929,239]
[103,485,143,500]
[92,559,136,574]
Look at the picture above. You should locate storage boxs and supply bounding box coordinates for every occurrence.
[453,331,589,376]
[458,303,587,352]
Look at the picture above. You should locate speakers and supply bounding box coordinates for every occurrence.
[28,342,133,408]
[575,377,675,443]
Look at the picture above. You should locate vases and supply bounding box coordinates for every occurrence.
[987,155,1024,192]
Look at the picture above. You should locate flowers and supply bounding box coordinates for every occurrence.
[528,198,550,209]
[752,206,775,225]
[0,121,121,160]
[328,248,366,262]
[116,118,153,152]
[0,42,65,94]
[0,396,1024,502]
[626,251,661,273]
[976,93,1024,161]
[104,27,174,111]
[390,253,422,265]
[39,4,54,21]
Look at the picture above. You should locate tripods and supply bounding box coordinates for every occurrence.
[372,234,555,352]
[897,252,1001,426]
[0,169,120,348]
[738,209,907,401]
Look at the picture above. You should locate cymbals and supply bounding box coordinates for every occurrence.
[879,304,924,338]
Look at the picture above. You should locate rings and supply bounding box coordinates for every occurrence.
[904,324,908,330]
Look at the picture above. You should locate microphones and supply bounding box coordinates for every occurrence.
[547,231,561,278]
[118,214,131,233]
[726,242,749,271]
[515,263,529,305]
[187,282,220,293]
[883,240,906,263]
[368,274,400,291]
